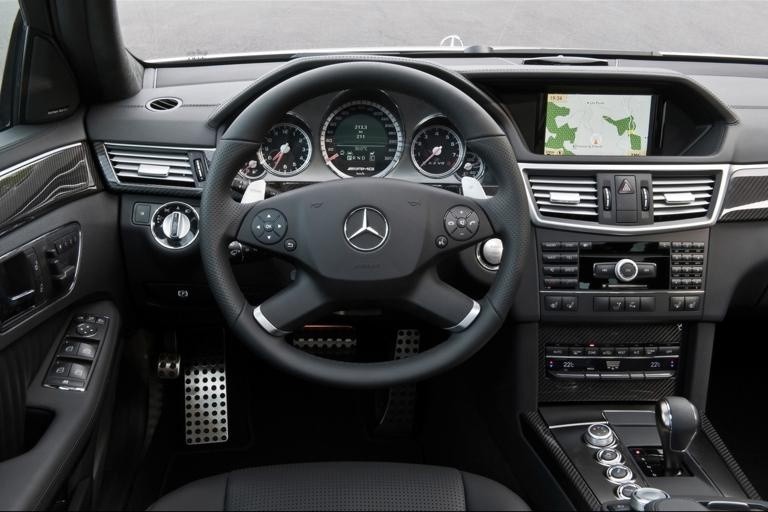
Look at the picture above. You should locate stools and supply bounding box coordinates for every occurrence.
[146,457,531,511]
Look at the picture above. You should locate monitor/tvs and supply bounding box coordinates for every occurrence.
[534,90,660,156]
